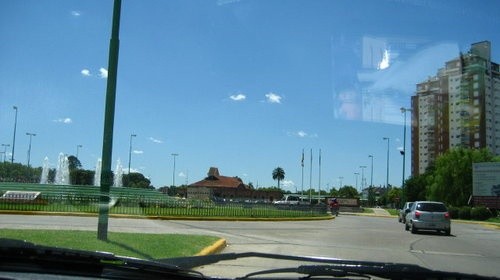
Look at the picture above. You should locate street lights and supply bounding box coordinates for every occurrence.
[368,155,373,208]
[383,137,390,207]
[11,106,18,164]
[354,172,360,196]
[26,132,36,167]
[360,165,367,207]
[399,107,407,208]
[128,134,137,173]
[76,144,83,159]
[171,153,179,197]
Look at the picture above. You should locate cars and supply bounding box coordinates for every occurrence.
[404,201,451,236]
[398,202,421,224]
[273,195,312,210]
